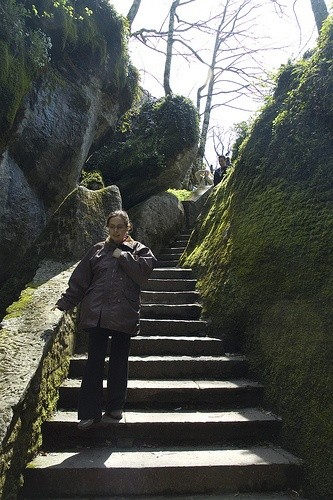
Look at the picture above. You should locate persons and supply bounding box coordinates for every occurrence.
[50,210,158,429]
[194,155,232,188]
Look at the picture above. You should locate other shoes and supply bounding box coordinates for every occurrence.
[78,416,101,429]
[106,410,122,420]
